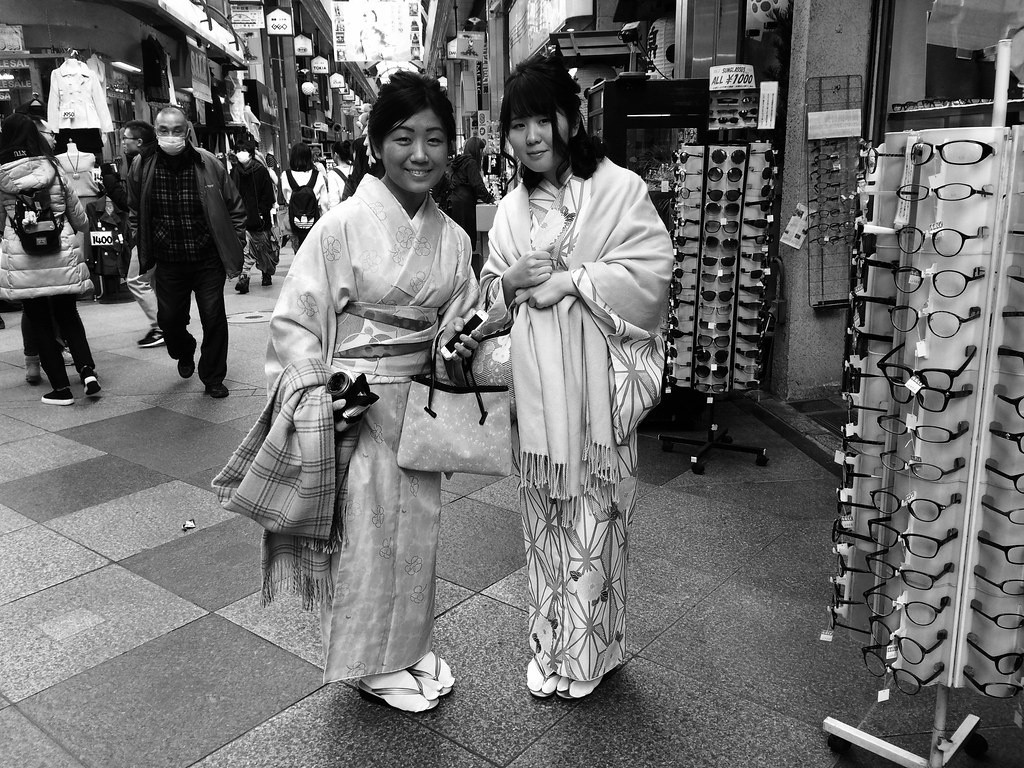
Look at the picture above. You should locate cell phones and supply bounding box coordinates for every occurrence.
[440,310,489,361]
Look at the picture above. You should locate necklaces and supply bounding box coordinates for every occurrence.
[67,151,80,180]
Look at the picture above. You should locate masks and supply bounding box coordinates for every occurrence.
[236,151,252,163]
[156,129,189,155]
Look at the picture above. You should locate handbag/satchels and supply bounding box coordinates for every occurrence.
[396,331,512,477]
[461,328,517,417]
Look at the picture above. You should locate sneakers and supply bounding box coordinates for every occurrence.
[138,328,164,347]
[205,382,229,398]
[178,338,196,378]
[79,366,101,395]
[41,386,75,406]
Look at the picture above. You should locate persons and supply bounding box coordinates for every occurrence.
[101,107,248,398]
[229,138,280,294]
[431,136,498,253]
[280,137,387,256]
[477,57,677,701]
[0,114,102,406]
[261,72,489,713]
[54,143,98,212]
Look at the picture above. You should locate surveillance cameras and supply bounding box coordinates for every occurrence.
[622,22,642,42]
[490,0,501,13]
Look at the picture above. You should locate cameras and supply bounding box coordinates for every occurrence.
[325,373,371,421]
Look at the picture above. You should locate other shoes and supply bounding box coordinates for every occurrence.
[234,281,249,294]
[261,277,273,286]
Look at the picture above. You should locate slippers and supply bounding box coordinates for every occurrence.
[357,672,440,713]
[527,653,560,698]
[404,654,451,696]
[556,664,617,699]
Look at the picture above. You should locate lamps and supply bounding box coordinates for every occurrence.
[296,67,315,96]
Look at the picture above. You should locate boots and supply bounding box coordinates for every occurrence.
[24,355,42,384]
[60,350,74,365]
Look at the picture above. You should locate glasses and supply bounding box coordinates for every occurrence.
[664,95,776,396]
[122,135,139,140]
[809,94,1024,699]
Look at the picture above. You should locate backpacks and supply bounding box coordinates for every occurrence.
[444,154,474,203]
[286,168,320,236]
[333,167,356,201]
[5,187,64,256]
